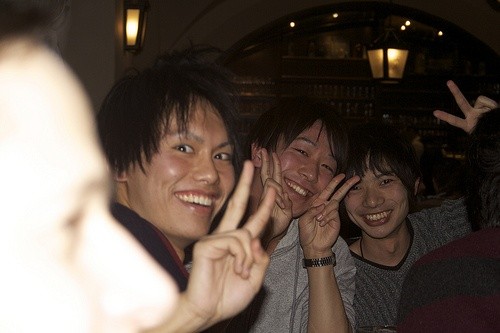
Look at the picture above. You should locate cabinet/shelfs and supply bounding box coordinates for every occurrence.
[210,56,464,164]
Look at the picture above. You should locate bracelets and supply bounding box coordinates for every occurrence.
[302,253,336,268]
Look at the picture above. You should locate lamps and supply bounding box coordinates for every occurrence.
[123,0,150,56]
[366,16,410,85]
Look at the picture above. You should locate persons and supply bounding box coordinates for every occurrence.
[99,53,500,333]
[0,0,185,333]
